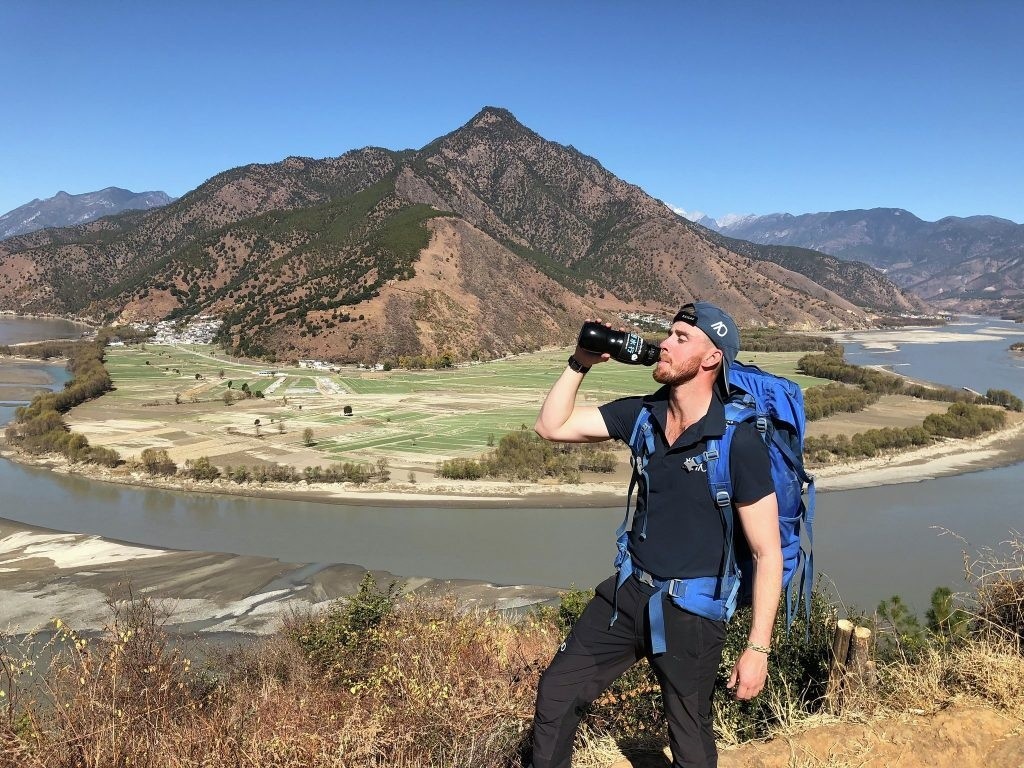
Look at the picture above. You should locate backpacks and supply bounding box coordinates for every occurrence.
[628,358,813,594]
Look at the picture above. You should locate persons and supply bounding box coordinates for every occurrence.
[526,301,785,768]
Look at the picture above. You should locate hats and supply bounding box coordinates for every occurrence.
[673,302,740,397]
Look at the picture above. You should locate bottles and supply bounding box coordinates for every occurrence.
[577,322,660,367]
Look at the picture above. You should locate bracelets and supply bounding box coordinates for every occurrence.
[567,354,590,374]
[746,640,772,655]
